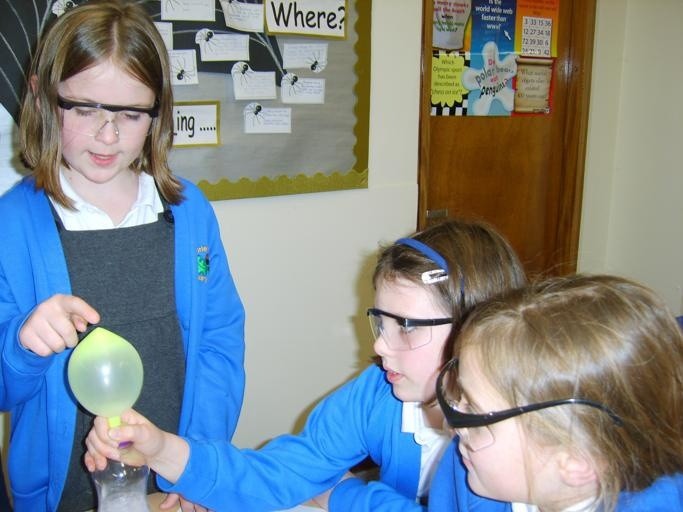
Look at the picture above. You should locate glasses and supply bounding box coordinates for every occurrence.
[368,308,454,353]
[56,92,155,140]
[435,357,622,452]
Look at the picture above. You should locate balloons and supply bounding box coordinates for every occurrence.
[64,324,148,431]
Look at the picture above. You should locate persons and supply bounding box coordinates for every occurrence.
[0,1,251,512]
[79,222,532,512]
[441,268,682,512]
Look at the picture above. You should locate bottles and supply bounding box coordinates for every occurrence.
[89,442,150,512]
